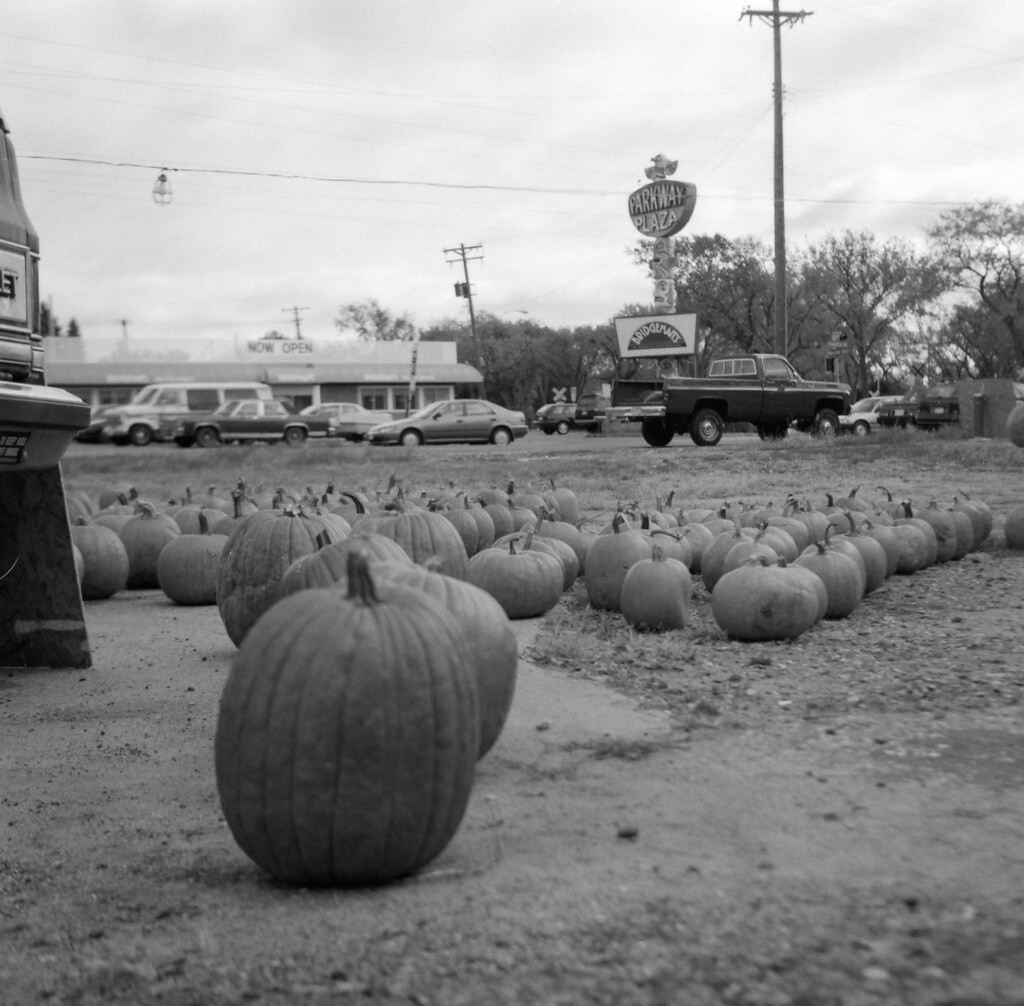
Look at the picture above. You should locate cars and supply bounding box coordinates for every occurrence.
[72,405,123,444]
[363,399,529,448]
[532,403,576,435]
[876,385,920,428]
[170,399,317,448]
[915,381,962,433]
[839,395,906,435]
[299,402,369,436]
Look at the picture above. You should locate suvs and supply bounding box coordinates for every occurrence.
[573,392,611,435]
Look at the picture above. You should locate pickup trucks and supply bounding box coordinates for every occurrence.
[605,352,852,448]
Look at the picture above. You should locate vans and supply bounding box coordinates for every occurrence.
[102,381,274,447]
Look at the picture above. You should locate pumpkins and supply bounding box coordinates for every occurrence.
[212,550,475,884]
[341,554,517,762]
[62,472,1024,642]
